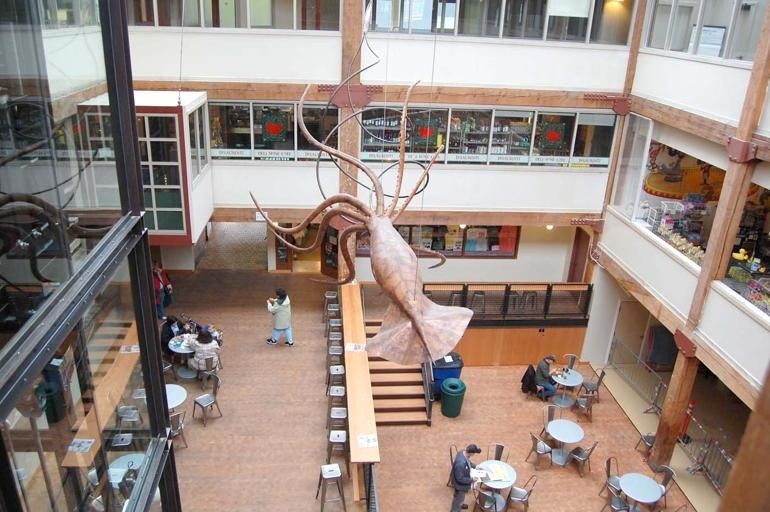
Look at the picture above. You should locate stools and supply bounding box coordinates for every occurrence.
[315,289,349,512]
[423,290,539,314]
[111,432,138,452]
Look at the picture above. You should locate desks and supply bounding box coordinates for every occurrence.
[167,333,198,379]
[143,382,189,413]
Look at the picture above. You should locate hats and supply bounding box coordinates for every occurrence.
[466,444,481,453]
[545,355,555,360]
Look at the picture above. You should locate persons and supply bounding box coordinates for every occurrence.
[185,329,221,380]
[179,313,203,337]
[206,324,219,341]
[265,288,294,348]
[161,315,192,356]
[150,259,173,321]
[449,444,485,511]
[531,354,559,403]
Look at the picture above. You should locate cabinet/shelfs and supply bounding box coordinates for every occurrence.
[360,126,513,156]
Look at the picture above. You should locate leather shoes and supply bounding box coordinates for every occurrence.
[462,504,468,509]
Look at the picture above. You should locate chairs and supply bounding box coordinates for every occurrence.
[106,391,144,429]
[170,353,223,450]
[446,353,678,512]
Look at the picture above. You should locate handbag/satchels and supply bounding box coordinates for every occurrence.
[163,285,175,308]
[447,478,454,487]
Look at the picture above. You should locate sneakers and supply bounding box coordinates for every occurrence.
[284,342,293,347]
[267,339,276,344]
[538,392,548,401]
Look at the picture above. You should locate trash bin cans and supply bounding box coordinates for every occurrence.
[440,377,466,419]
[433,352,464,401]
